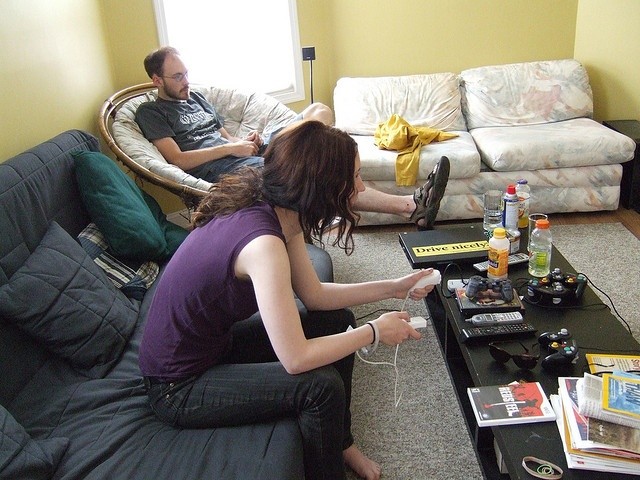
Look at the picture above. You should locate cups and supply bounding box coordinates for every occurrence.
[527,213,548,252]
[482,190,504,232]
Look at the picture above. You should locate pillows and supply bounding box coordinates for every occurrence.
[75,224,160,294]
[1,221,141,378]
[0,405,70,479]
[70,150,191,263]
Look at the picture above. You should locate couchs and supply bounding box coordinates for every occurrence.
[0,130,334,480]
[331,57,637,226]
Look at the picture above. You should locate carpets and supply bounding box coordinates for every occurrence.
[302,221,640,476]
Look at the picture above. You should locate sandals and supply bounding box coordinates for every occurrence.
[409,156,450,231]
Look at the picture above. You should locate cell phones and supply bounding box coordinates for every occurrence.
[465,311,524,327]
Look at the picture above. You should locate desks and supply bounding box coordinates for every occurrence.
[422,240,640,480]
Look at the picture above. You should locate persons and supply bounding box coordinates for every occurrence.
[136,46,451,231]
[138,120,435,477]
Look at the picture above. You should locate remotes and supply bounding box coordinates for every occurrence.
[473,252,530,272]
[459,322,538,343]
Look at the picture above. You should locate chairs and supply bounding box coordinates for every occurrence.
[99,79,345,235]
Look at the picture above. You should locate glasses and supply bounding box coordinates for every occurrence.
[157,71,190,82]
[488,339,542,371]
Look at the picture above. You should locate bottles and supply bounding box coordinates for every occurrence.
[487,228,510,281]
[528,219,553,277]
[516,179,531,228]
[503,185,519,229]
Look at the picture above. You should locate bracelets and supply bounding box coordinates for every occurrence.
[366,320,380,352]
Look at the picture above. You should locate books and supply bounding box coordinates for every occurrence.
[399,226,493,270]
[550,370,640,476]
